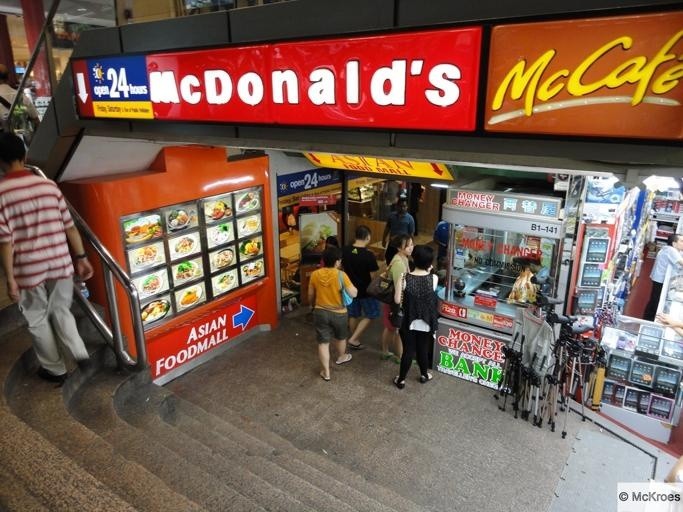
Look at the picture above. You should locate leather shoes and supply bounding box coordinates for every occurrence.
[77,359,92,373]
[37,364,69,383]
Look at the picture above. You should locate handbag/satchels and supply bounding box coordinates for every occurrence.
[366,259,400,305]
[338,270,353,306]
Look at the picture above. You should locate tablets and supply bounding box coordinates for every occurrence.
[577,236,683,424]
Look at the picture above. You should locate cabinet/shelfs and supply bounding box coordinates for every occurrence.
[585,195,683,315]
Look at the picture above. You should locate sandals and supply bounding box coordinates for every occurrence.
[420,372,434,383]
[393,375,405,389]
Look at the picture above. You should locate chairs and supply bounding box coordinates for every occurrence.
[285,253,301,280]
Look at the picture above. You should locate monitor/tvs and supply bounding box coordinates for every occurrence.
[16,68,25,75]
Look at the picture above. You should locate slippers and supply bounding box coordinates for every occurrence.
[319,370,331,381]
[336,353,352,365]
[347,342,368,350]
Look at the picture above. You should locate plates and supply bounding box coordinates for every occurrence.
[123,188,265,327]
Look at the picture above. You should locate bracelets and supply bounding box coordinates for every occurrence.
[73,252,87,260]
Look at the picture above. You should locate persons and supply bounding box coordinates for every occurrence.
[319,235,343,268]
[379,233,414,365]
[0,132,95,382]
[525,252,551,287]
[657,312,683,349]
[377,178,450,297]
[511,256,538,303]
[306,245,358,381]
[0,64,41,134]
[339,224,381,350]
[642,233,683,323]
[392,244,439,389]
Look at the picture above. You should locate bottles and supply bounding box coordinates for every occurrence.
[79,282,89,302]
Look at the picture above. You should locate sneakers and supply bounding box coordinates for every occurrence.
[381,350,401,365]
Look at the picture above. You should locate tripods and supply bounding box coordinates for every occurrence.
[494,283,586,439]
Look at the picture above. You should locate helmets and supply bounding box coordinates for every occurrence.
[434,221,449,243]
